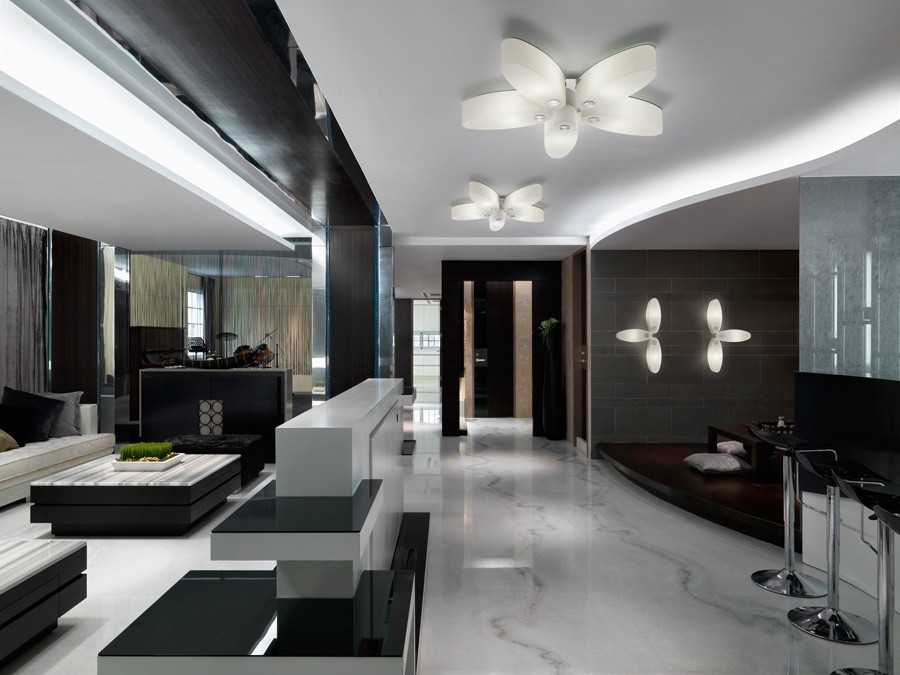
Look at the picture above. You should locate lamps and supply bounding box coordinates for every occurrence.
[461,38,664,159]
[707,298,752,373]
[615,297,661,374]
[450,181,545,232]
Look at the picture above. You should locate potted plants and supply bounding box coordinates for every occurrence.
[539,317,563,350]
[115,441,185,471]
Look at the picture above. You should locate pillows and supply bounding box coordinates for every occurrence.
[34,391,84,438]
[717,441,747,456]
[684,453,755,475]
[0,429,19,452]
[2,386,65,442]
[0,404,38,447]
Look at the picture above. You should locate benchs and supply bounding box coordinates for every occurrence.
[164,434,264,493]
[0,539,87,662]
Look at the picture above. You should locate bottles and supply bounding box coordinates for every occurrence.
[777,417,785,427]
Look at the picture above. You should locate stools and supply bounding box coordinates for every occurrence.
[787,447,892,645]
[747,426,826,598]
[830,469,900,675]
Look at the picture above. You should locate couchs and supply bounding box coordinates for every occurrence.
[0,384,115,508]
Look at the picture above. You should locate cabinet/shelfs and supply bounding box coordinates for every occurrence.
[97,377,431,675]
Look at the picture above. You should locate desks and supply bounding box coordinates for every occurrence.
[707,421,787,476]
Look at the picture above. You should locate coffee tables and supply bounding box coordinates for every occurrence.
[29,453,243,537]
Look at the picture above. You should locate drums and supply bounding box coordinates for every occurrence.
[233,345,253,357]
[253,343,275,365]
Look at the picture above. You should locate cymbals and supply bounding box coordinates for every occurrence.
[215,332,238,341]
[259,327,279,343]
